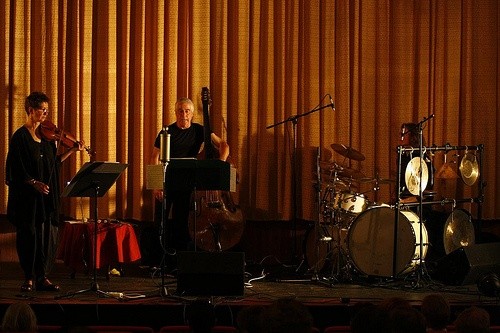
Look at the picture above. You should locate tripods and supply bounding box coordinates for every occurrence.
[159,160,229,288]
[310,127,446,292]
[57,161,128,303]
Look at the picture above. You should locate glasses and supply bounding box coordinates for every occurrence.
[39,107,49,113]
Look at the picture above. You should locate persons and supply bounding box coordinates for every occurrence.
[151,97,229,277]
[349,298,426,333]
[0,303,37,333]
[455,307,490,333]
[259,298,313,333]
[395,123,434,225]
[421,295,453,333]
[6,92,85,291]
[239,305,267,333]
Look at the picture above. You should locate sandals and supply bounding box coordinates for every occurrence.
[22,282,32,291]
[36,276,59,291]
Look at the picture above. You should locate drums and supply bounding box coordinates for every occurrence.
[346,205,428,279]
[333,190,369,215]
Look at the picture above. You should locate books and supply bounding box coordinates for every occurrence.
[60,162,119,197]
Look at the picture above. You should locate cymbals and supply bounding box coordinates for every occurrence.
[330,144,365,161]
[314,161,366,179]
[359,177,395,184]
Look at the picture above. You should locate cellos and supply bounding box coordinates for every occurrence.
[187,86,244,252]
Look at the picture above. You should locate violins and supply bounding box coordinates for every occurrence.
[38,120,97,157]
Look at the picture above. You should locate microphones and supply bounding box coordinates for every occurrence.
[177,249,247,296]
[438,242,500,287]
[329,96,335,111]
[401,124,406,141]
